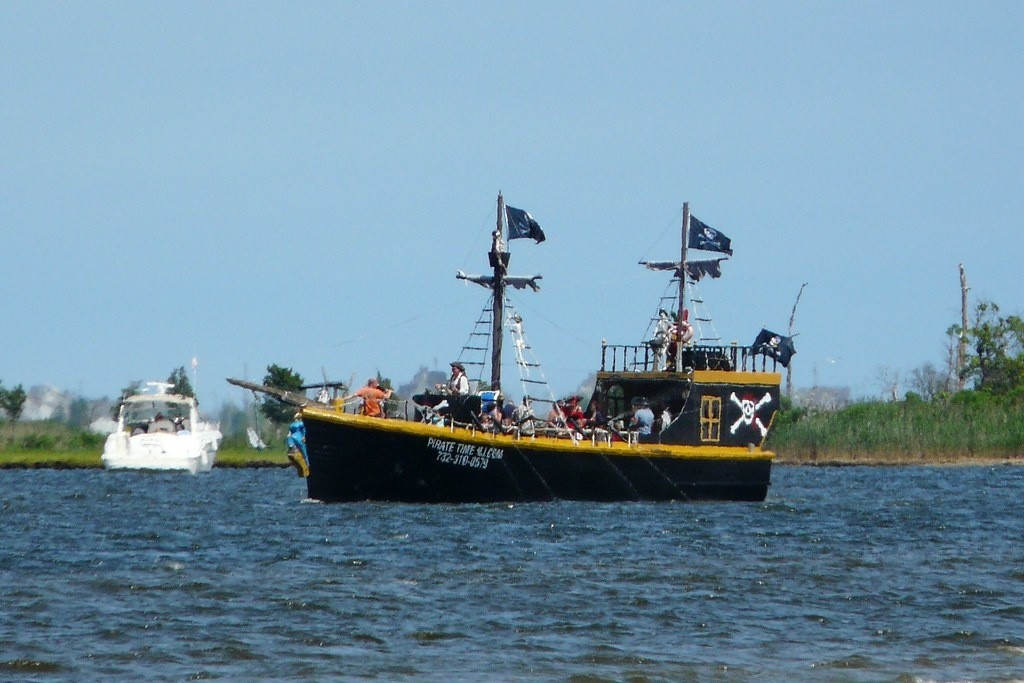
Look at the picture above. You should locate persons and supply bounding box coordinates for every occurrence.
[177,425,191,436]
[285,412,310,468]
[547,395,654,444]
[435,362,536,438]
[132,426,144,436]
[155,412,166,422]
[653,308,694,370]
[346,377,394,418]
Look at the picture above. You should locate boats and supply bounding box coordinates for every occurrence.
[100,380,227,476]
[225,186,786,508]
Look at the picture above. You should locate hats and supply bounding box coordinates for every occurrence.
[450,362,465,371]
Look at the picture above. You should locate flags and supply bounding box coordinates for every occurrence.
[687,215,733,256]
[749,328,796,366]
[506,205,546,245]
